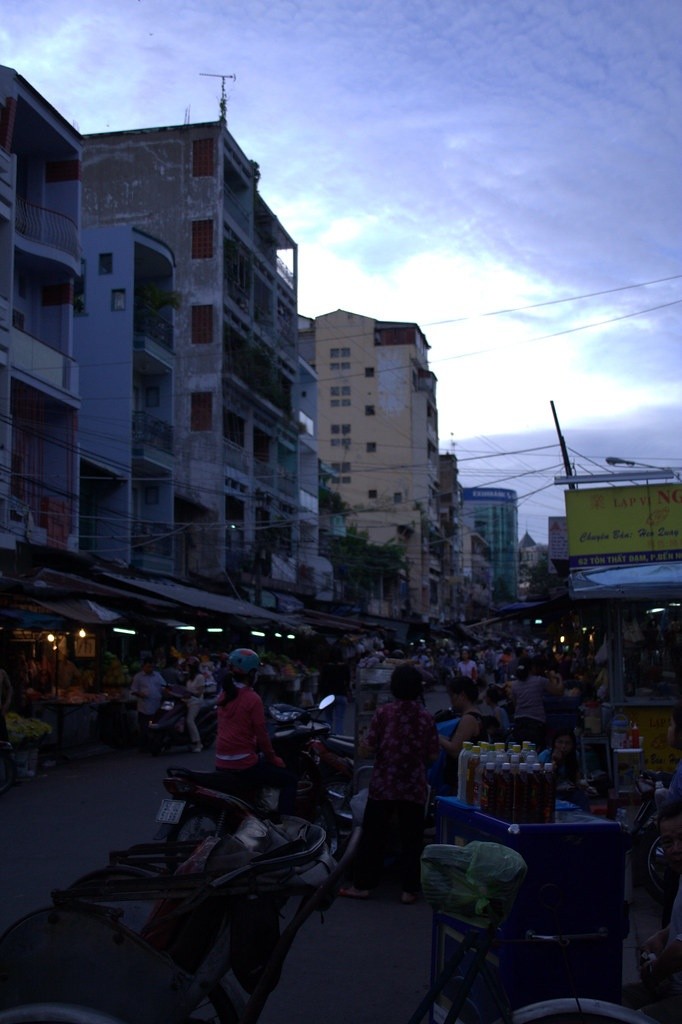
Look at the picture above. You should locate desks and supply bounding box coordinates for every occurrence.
[579,736,611,784]
[27,699,141,754]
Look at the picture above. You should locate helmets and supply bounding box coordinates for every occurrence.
[184,656,200,667]
[228,648,264,674]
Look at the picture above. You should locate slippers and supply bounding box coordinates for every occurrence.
[337,888,368,900]
[404,893,419,905]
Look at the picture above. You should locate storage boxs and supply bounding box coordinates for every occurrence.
[428,794,631,1024]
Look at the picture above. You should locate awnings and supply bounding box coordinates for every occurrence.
[0,567,384,633]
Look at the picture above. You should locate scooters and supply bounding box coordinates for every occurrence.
[0,692,682,1024]
[140,684,226,758]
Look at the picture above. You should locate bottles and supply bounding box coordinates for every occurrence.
[631,723,640,748]
[610,714,629,749]
[455,740,558,824]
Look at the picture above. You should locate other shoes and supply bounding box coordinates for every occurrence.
[193,744,203,753]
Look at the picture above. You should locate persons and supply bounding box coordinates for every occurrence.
[374,643,477,683]
[180,656,205,752]
[0,670,14,743]
[472,640,589,791]
[130,657,166,751]
[337,665,439,904]
[622,702,682,1024]
[320,648,351,735]
[439,675,484,794]
[161,656,183,684]
[213,648,309,770]
